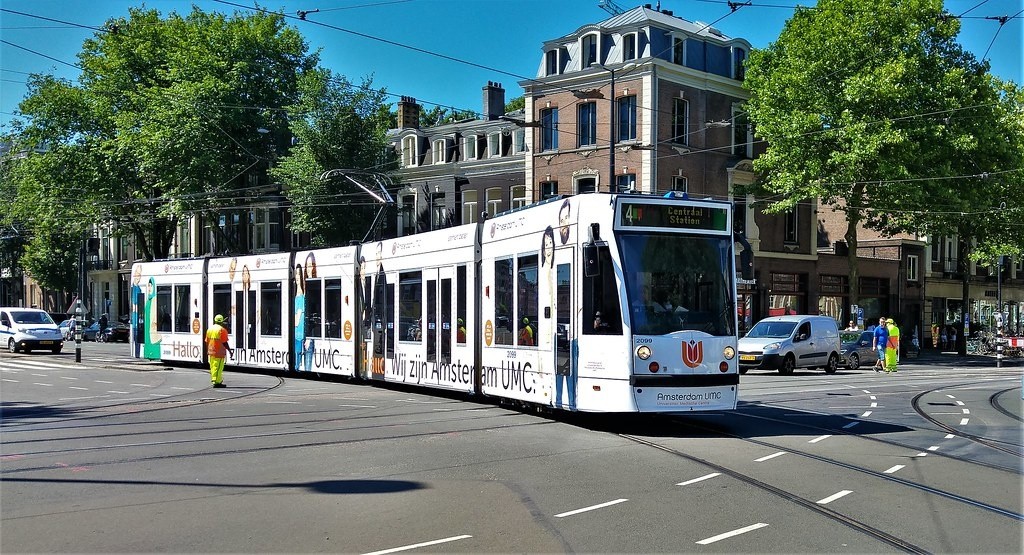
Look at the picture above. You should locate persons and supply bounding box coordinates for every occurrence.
[131,265,162,361]
[632,285,665,313]
[844,320,858,332]
[866,317,900,373]
[204,314,233,388]
[940,326,957,351]
[541,199,579,411]
[360,242,386,334]
[229,258,251,290]
[294,252,317,369]
[518,318,533,346]
[457,318,466,343]
[66,315,76,334]
[97,314,108,338]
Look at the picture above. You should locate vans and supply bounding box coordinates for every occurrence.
[738,314,846,373]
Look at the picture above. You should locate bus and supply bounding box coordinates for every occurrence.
[127,172,756,416]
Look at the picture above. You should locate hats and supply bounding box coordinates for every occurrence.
[214,314,223,323]
[457,318,463,325]
[885,319,894,324]
[523,318,529,324]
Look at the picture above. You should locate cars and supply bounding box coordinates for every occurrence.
[80,320,129,343]
[838,330,879,370]
[0,308,64,353]
[56,319,89,341]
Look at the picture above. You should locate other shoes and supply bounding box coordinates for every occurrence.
[873,367,879,373]
[213,383,226,388]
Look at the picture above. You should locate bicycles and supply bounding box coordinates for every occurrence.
[977,333,1023,360]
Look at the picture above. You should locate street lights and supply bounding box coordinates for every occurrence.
[80,228,101,336]
[591,61,616,192]
